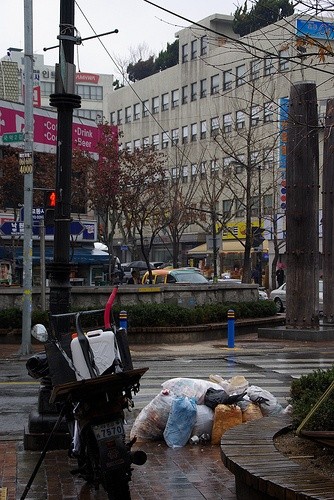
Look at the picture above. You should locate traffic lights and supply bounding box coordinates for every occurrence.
[252,226,266,247]
[43,190,56,228]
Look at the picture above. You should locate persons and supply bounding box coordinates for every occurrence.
[0,263,10,279]
[129,271,138,284]
[251,264,262,287]
[276,268,284,287]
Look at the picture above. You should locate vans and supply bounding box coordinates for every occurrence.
[141,269,208,284]
[115,262,134,284]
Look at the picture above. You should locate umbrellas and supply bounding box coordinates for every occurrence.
[277,263,285,269]
[129,261,155,278]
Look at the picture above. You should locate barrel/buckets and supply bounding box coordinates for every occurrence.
[69,329,123,381]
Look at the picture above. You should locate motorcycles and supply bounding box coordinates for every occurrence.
[26,323,147,500]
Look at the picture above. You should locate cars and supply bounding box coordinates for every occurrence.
[270,281,324,313]
[210,278,268,300]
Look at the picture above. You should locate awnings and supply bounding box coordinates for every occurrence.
[188,240,269,255]
